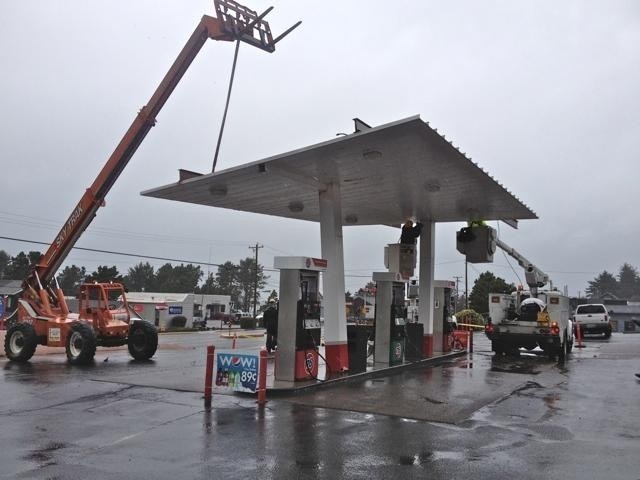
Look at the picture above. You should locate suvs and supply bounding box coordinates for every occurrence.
[572,302,613,339]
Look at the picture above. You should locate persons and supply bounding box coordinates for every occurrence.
[400,220,422,244]
[262,300,277,353]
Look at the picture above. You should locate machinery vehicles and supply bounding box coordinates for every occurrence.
[0,0,304,368]
[453,223,575,365]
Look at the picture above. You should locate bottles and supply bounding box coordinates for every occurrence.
[216,367,240,388]
